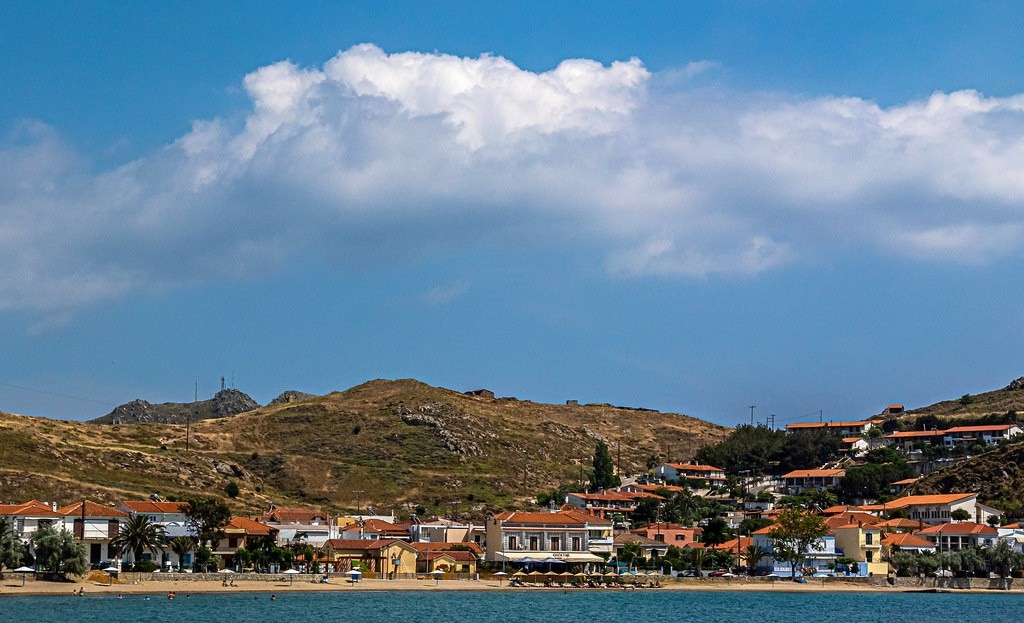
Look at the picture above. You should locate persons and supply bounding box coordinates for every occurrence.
[230,580,239,587]
[513,570,661,588]
[222,579,229,587]
[73,586,277,601]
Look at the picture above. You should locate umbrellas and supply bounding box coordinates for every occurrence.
[766,573,780,588]
[345,569,361,587]
[282,568,299,586]
[815,574,829,587]
[217,568,236,587]
[102,567,122,586]
[540,556,566,571]
[429,569,446,587]
[493,571,508,587]
[14,565,36,587]
[722,572,736,585]
[513,555,543,573]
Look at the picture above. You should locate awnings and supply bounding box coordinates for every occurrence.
[500,553,604,562]
